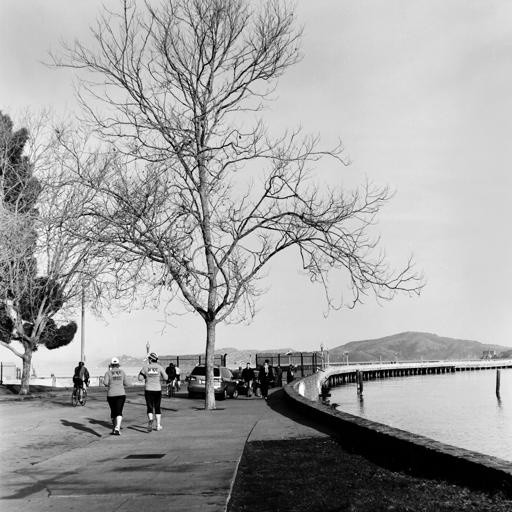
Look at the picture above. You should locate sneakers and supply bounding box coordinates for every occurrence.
[148,419,155,431]
[156,425,163,431]
[113,427,121,434]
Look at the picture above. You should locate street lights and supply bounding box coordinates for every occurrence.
[146,340,150,355]
[394,352,398,364]
[326,346,330,368]
[320,341,325,369]
[342,352,345,364]
[345,348,349,365]
[78,276,91,363]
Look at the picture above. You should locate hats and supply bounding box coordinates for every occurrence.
[111,358,120,364]
[149,352,157,360]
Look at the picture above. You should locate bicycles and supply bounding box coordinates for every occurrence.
[71,380,89,407]
[175,378,181,393]
[166,380,176,398]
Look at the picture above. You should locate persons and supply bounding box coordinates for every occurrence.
[138,352,169,431]
[173,366,181,388]
[104,357,128,434]
[241,359,295,399]
[165,363,176,394]
[72,361,89,401]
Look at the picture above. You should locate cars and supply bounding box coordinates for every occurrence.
[185,362,280,400]
